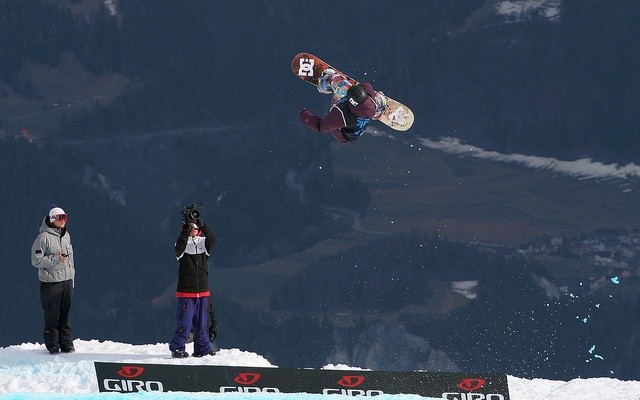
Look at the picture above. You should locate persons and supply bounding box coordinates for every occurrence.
[299,69,378,144]
[31,207,78,354]
[168,215,218,358]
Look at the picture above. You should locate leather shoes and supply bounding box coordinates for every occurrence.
[182,224,191,234]
[196,218,205,228]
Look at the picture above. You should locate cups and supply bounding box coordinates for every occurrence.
[190,222,199,230]
[52,214,69,223]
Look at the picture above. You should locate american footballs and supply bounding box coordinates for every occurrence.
[291,52,414,132]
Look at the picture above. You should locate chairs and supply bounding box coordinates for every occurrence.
[172,350,185,359]
[192,350,216,357]
[49,347,59,354]
[61,345,75,352]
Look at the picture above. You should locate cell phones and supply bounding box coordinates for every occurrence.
[181,201,203,227]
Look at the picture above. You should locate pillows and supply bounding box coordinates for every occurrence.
[348,86,368,107]
[48,206,66,223]
